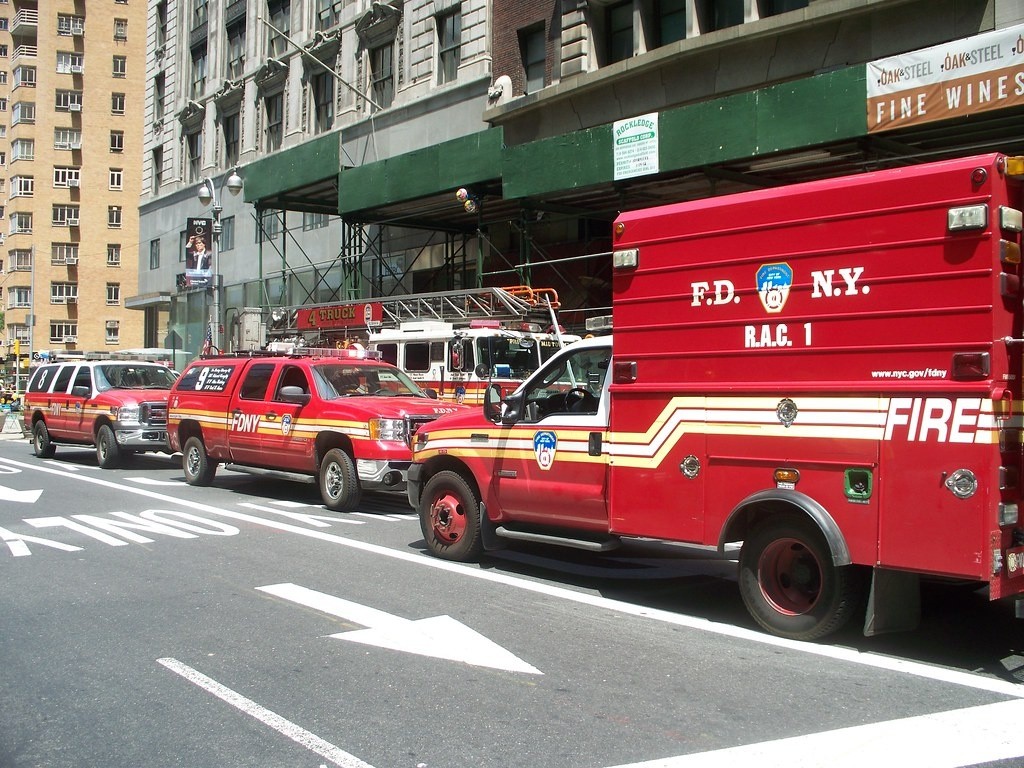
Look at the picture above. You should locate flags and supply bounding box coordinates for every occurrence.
[202,322,212,354]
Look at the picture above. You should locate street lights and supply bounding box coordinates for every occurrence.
[197,164,244,357]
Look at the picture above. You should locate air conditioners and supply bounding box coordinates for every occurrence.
[65,336,75,342]
[106,321,119,328]
[71,142,81,149]
[69,104,80,112]
[69,219,80,226]
[66,297,77,303]
[67,179,79,186]
[72,65,82,74]
[65,258,78,265]
[73,28,83,36]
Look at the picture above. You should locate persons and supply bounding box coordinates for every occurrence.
[7,384,14,392]
[186,236,211,270]
[0,385,5,390]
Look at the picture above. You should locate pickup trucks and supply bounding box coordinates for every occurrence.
[166,342,472,511]
[23,361,177,468]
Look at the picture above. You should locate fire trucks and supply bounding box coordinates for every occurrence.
[254,284,597,411]
[407,153,1024,638]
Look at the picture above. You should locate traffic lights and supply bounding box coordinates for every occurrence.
[13,338,19,355]
